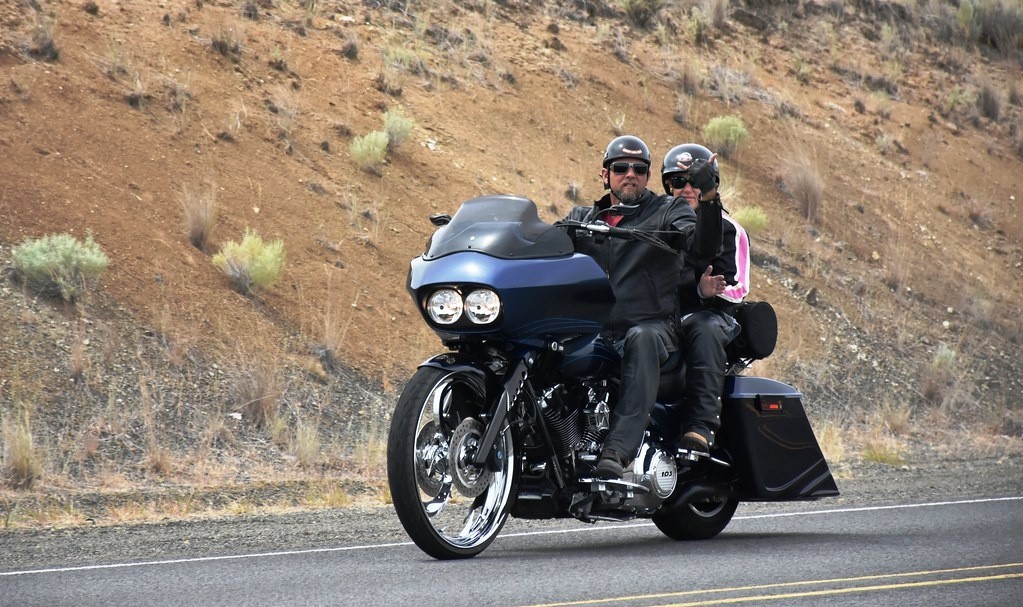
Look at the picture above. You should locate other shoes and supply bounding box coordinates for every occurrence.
[679,431,714,454]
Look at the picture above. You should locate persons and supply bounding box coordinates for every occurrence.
[542,134,697,482]
[659,141,754,458]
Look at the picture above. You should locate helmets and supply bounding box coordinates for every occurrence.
[602,135,651,168]
[661,142,720,195]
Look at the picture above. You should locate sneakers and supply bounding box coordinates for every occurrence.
[596,449,625,478]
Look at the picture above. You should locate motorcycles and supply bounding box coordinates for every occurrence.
[386,194,842,562]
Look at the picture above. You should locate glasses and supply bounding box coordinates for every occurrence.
[668,175,700,189]
[609,161,649,176]
